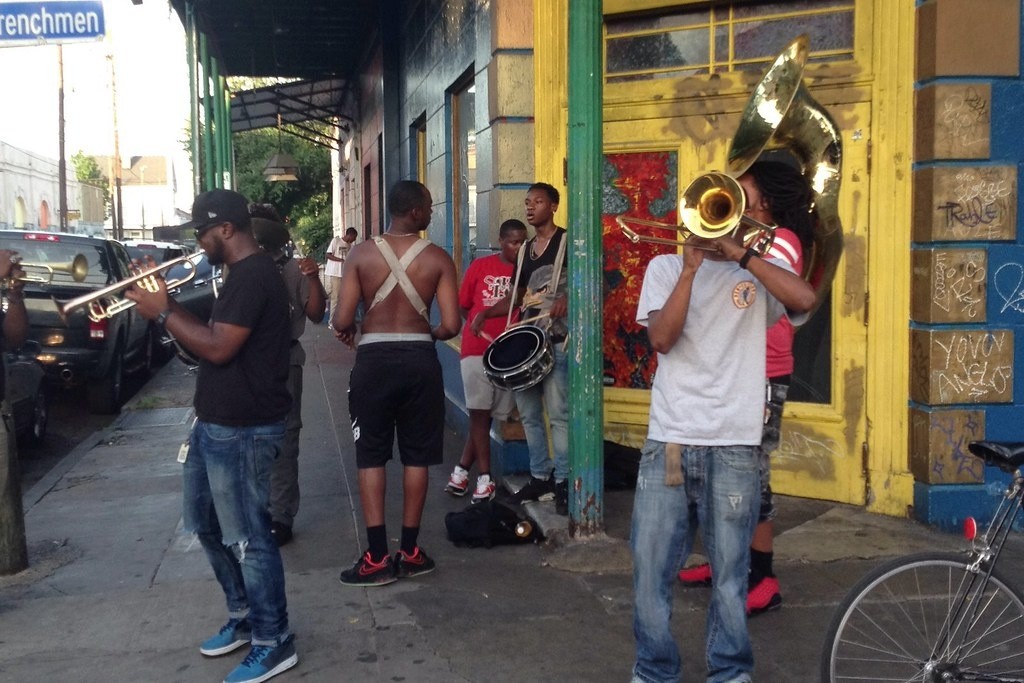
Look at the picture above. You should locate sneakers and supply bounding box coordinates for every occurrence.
[506,467,556,504]
[444,465,469,496]
[555,478,568,515]
[678,562,712,587]
[222,634,299,683]
[471,474,496,504]
[393,546,435,577]
[745,575,782,618]
[199,618,252,656]
[339,549,398,586]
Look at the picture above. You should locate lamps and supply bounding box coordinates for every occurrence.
[263,90,299,167]
[264,175,297,181]
[262,167,297,175]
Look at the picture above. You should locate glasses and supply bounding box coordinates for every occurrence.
[194,222,236,240]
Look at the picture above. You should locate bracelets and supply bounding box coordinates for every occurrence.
[6,294,24,305]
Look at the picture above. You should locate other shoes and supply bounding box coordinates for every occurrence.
[273,520,293,547]
[328,325,334,330]
[632,675,646,683]
[725,672,752,683]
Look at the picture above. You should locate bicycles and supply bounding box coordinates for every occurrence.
[820,439,1024,683]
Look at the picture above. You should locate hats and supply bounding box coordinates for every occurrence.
[177,189,249,230]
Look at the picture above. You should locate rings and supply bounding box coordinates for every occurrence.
[10,255,17,264]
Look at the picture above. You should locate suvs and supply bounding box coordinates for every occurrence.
[0,229,154,414]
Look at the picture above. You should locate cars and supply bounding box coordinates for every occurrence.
[117,237,199,279]
[0,340,50,447]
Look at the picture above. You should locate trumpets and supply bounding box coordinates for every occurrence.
[49,247,209,330]
[0,252,90,292]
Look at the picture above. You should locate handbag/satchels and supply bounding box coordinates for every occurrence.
[445,486,546,549]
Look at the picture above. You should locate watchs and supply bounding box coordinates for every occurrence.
[739,248,760,270]
[157,309,173,326]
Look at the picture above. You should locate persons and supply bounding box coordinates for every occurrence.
[331,181,463,586]
[472,182,568,517]
[326,228,358,330]
[123,190,326,683]
[444,218,529,505]
[0,249,31,577]
[628,161,818,683]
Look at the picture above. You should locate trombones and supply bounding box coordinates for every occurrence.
[614,171,776,262]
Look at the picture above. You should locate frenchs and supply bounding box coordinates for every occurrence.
[723,34,845,333]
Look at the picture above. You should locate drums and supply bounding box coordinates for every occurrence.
[481,325,555,392]
